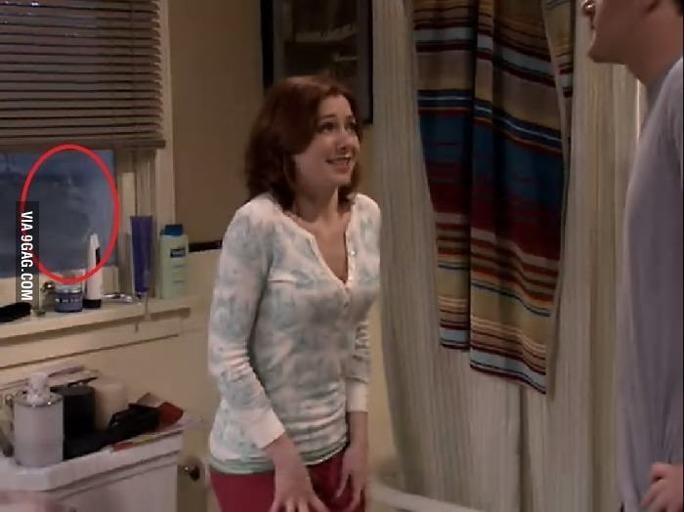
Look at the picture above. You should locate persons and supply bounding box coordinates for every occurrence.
[579,0,683,512]
[207,74,384,512]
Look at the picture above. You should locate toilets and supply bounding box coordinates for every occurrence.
[0,434,181,512]
